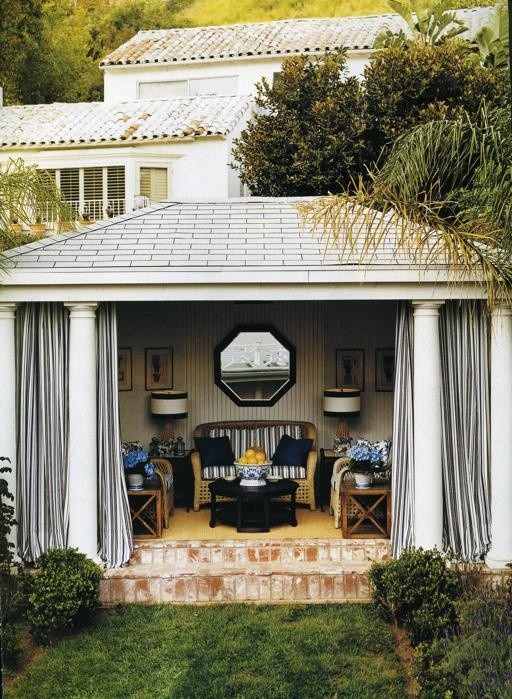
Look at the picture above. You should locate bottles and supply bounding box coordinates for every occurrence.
[150,437,160,456]
[175,437,186,456]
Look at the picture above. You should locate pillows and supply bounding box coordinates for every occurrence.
[190,433,315,470]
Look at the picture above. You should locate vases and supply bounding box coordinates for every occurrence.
[125,472,145,491]
[353,472,373,490]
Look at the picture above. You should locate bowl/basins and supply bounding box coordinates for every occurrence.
[233,460,274,487]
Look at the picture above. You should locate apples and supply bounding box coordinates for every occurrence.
[239,449,268,464]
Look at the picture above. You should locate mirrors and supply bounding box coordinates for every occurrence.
[212,322,297,409]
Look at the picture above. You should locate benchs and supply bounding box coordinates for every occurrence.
[188,419,319,511]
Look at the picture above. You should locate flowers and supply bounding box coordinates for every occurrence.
[346,438,389,471]
[123,437,175,481]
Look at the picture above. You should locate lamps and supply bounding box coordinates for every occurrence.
[148,388,188,437]
[322,386,361,442]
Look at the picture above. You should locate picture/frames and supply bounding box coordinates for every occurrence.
[333,348,397,393]
[114,345,174,392]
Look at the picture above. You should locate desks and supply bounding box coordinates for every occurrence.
[339,481,391,540]
[318,448,343,513]
[127,486,164,540]
[147,449,195,507]
